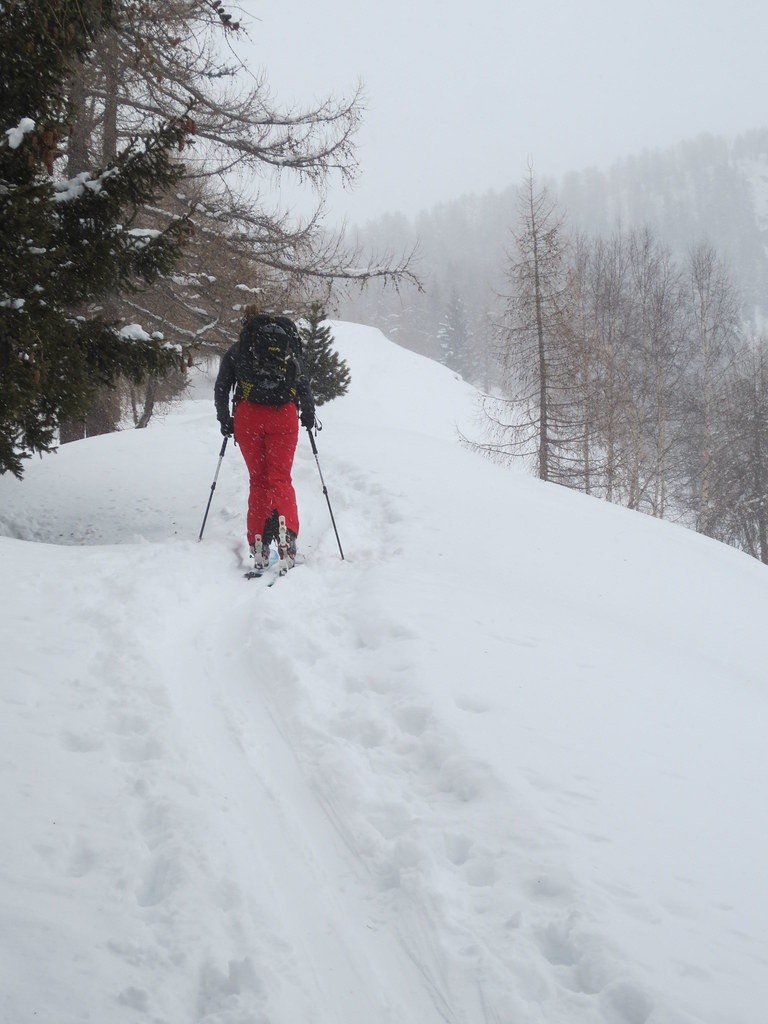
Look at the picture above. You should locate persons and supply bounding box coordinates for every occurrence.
[214,311,317,570]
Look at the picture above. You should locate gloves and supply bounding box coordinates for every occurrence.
[303,410,314,431]
[220,416,233,438]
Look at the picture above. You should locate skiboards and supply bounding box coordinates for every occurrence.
[245,551,304,588]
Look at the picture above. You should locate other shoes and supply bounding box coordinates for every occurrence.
[278,531,297,559]
[253,545,269,568]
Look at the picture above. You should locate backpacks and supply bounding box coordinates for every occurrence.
[239,314,301,405]
